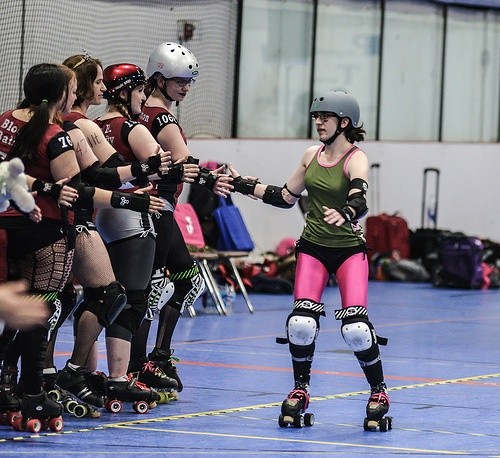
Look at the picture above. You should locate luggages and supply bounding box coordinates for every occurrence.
[366,162,408,257]
[414,168,443,256]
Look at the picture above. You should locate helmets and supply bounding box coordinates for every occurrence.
[309,90,360,127]
[103,63,146,98]
[145,42,199,79]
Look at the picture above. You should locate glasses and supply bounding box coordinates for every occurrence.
[173,78,195,87]
[311,113,336,120]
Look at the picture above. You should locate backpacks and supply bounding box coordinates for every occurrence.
[367,226,500,288]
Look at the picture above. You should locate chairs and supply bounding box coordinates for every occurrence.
[173,203,254,315]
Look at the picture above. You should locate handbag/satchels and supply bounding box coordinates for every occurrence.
[174,203,205,248]
[215,194,253,252]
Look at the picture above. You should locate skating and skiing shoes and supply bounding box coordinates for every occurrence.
[363,384,393,431]
[0,348,183,433]
[278,380,314,428]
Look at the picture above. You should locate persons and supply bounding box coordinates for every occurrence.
[92,63,234,415]
[63,54,200,417]
[216,91,394,433]
[130,42,234,404]
[0,56,172,433]
